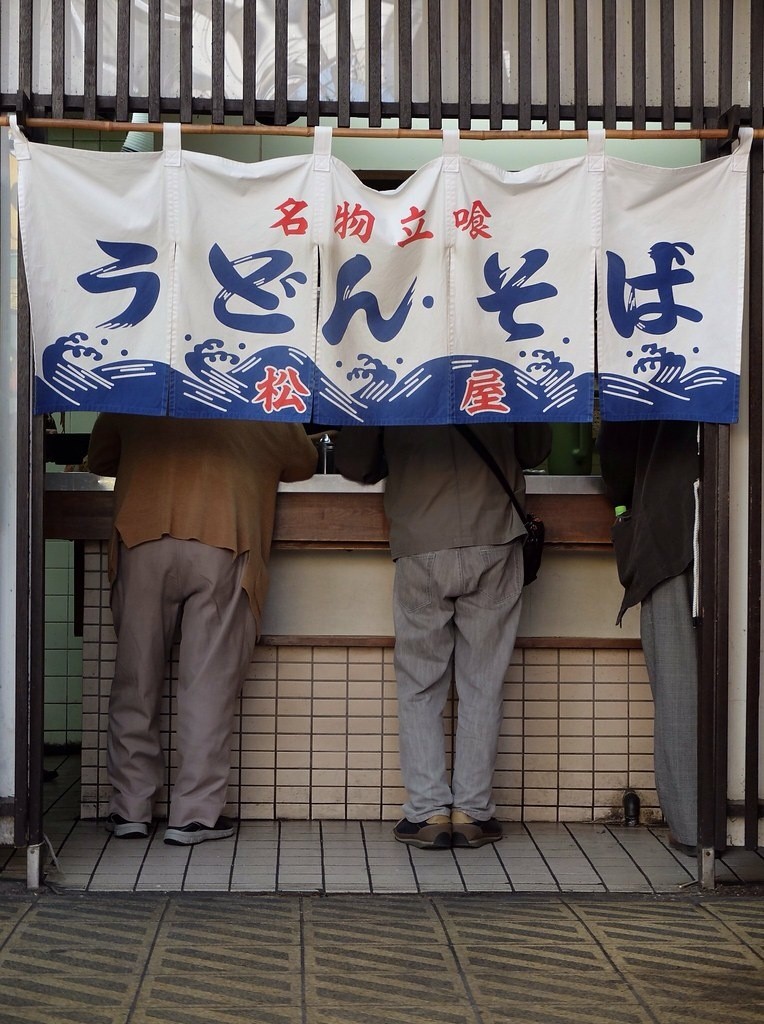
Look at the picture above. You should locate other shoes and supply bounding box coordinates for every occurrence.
[668,836,697,856]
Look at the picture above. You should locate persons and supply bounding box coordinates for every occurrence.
[88,412,318,844]
[335,423,553,849]
[597,421,701,857]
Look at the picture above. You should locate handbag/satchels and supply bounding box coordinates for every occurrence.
[523,514,543,586]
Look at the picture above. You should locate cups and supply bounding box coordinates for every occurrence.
[548,422,592,475]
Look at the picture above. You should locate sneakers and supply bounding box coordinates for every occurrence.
[164,814,233,844]
[104,813,147,837]
[452,817,502,847]
[393,817,452,848]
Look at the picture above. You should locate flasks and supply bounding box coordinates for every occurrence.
[316,434,335,474]
